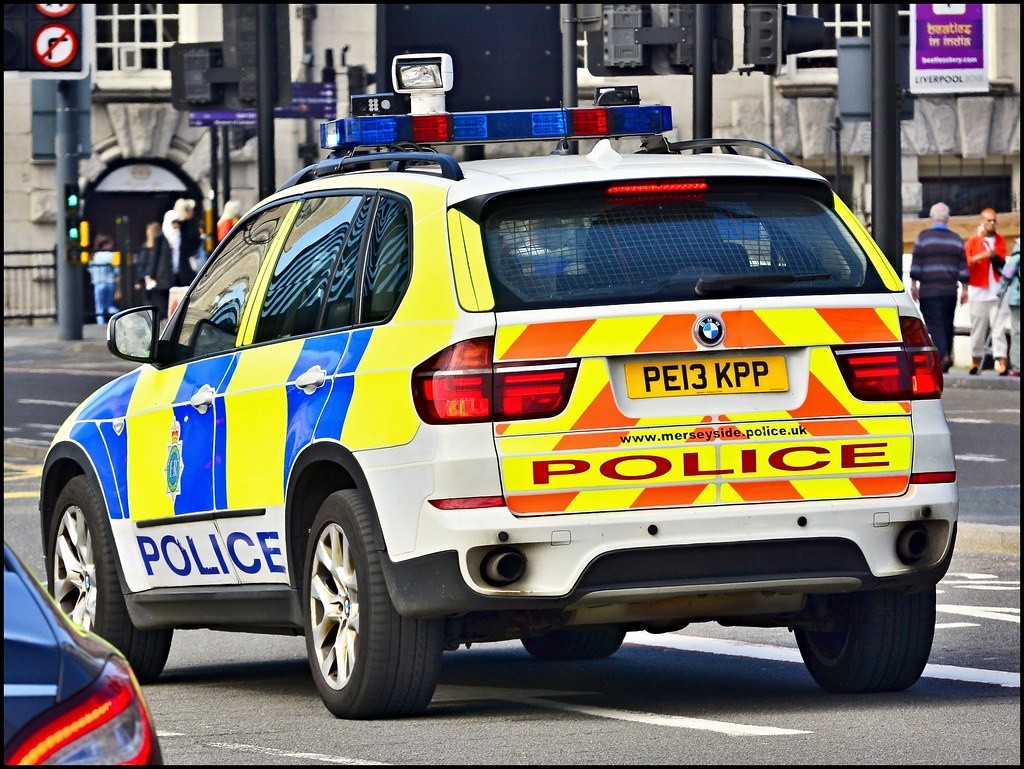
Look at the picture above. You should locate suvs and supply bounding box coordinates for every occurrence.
[37,100,964,726]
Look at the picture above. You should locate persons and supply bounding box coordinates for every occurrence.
[964,209,1008,376]
[133,198,205,321]
[89,236,120,325]
[996,239,1020,376]
[217,200,242,244]
[910,203,969,374]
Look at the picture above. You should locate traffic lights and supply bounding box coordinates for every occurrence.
[62,183,82,243]
[742,4,828,76]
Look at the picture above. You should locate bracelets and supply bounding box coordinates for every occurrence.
[911,288,917,291]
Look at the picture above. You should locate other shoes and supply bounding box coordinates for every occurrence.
[1000,369,1008,376]
[942,359,954,373]
[970,368,977,374]
